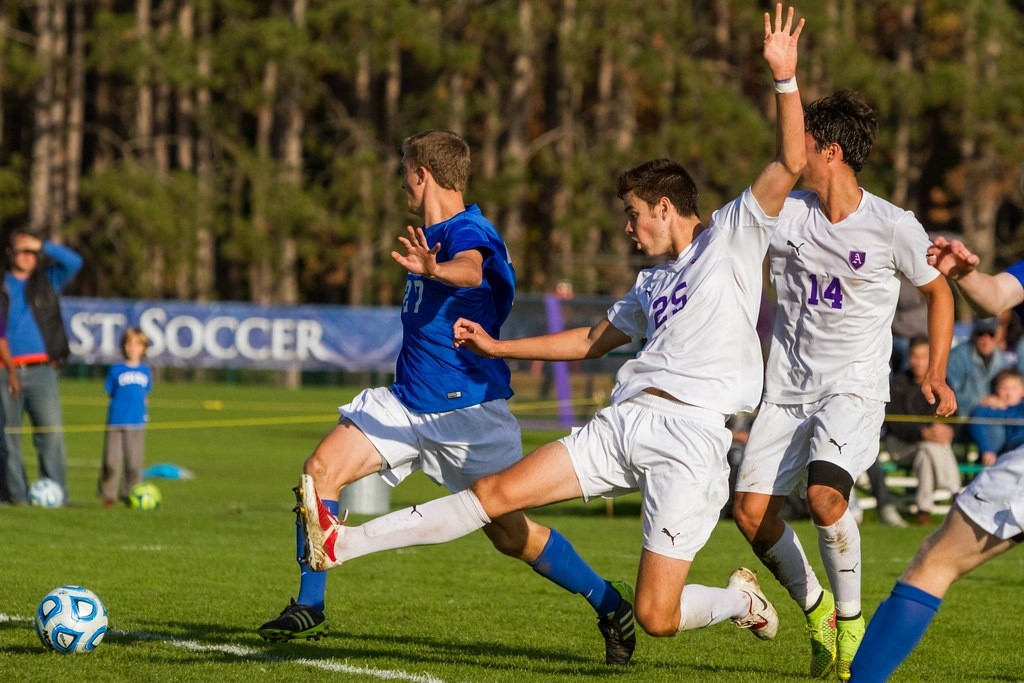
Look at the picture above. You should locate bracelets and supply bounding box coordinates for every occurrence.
[774,75,798,94]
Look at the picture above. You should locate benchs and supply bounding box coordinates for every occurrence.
[853,460,989,515]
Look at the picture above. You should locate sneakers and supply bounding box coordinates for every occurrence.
[291,474,349,572]
[597,579,636,668]
[804,589,838,679]
[726,566,778,640]
[258,597,328,643]
[836,614,866,681]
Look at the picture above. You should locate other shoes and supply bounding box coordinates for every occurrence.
[918,513,930,524]
[880,509,907,528]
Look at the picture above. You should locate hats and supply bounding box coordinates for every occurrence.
[972,314,998,331]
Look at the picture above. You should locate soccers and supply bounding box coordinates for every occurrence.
[28,477,65,509]
[35,585,109,653]
[128,483,162,511]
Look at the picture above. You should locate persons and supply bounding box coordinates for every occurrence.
[846,237,1024,683]
[970,368,1024,465]
[735,89,958,681]
[945,315,1009,417]
[0,232,84,508]
[885,332,961,524]
[0,321,20,397]
[257,131,638,666]
[292,2,808,640]
[96,327,153,508]
